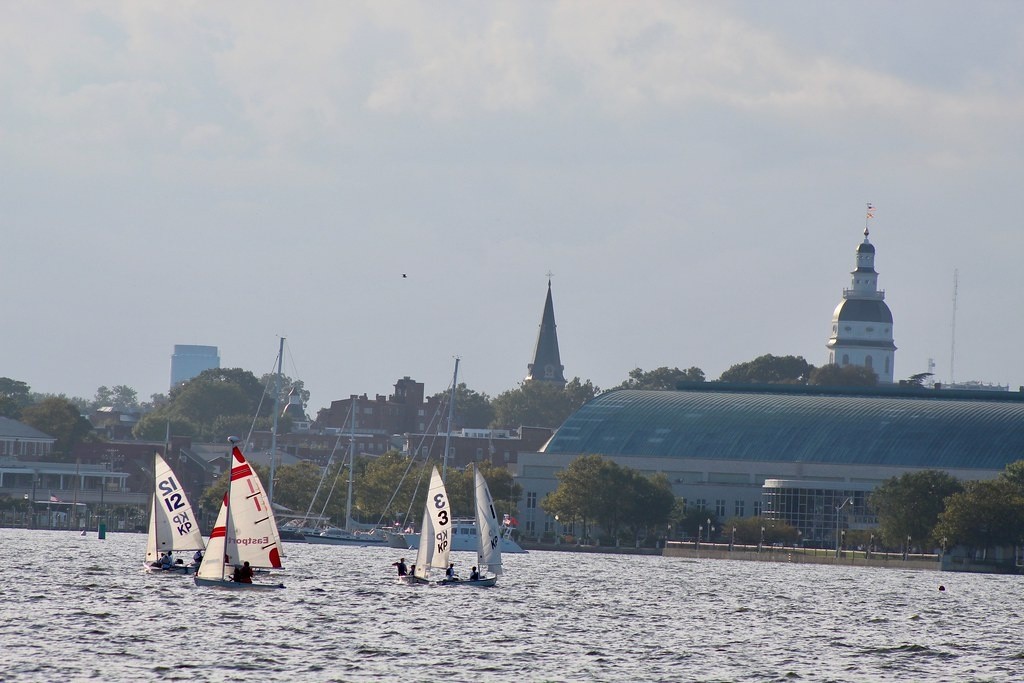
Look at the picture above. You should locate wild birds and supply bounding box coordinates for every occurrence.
[402,273,407,278]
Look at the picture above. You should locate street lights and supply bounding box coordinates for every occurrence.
[24,493,28,527]
[555,515,559,547]
[836,496,853,558]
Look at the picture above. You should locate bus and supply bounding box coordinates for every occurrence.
[33,501,87,529]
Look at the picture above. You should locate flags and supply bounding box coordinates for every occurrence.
[51,494,60,502]
[867,202,878,219]
[510,510,525,526]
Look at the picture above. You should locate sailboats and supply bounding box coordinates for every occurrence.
[242,337,528,554]
[142,436,288,588]
[395,465,504,588]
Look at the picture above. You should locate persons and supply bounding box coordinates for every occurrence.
[159,550,229,570]
[469,566,478,580]
[446,563,457,581]
[392,558,407,576]
[240,561,254,584]
[408,565,416,575]
